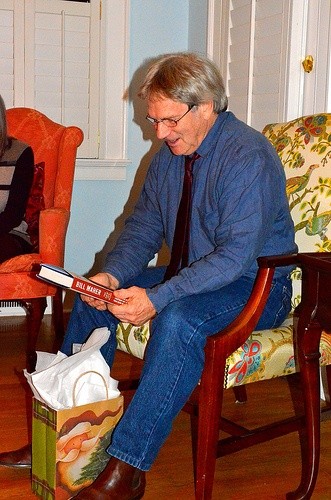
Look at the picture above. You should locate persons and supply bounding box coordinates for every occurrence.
[0,94,35,263]
[0,52,299,500]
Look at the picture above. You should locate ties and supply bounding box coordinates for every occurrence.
[163,151,202,283]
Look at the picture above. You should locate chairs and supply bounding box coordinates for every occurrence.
[114,112,331,500]
[0,107,84,370]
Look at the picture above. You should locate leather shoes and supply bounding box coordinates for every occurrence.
[0,444,32,468]
[70,456,146,500]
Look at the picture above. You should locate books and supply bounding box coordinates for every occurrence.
[36,263,127,307]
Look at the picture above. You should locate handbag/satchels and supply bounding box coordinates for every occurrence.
[23,327,121,411]
[23,372,123,500]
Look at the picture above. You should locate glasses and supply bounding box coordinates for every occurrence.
[145,104,195,127]
